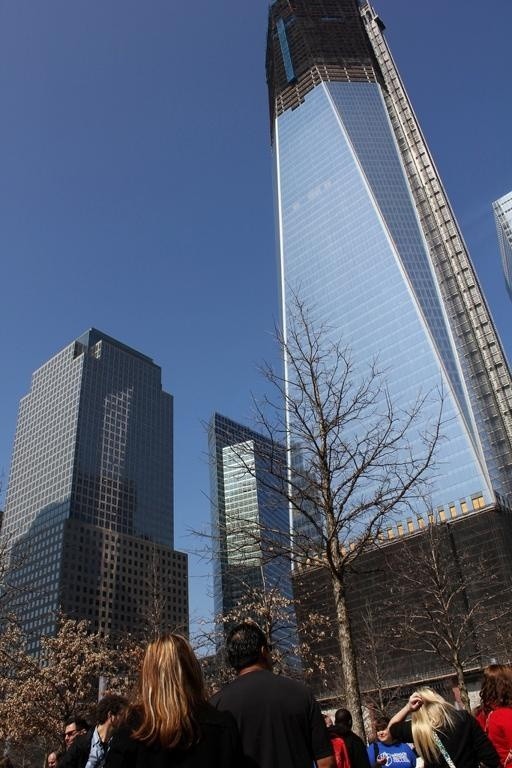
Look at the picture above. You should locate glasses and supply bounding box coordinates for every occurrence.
[64,728,79,737]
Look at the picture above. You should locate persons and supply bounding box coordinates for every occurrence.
[322,664,512,768]
[103,632,236,767]
[49,694,131,768]
[206,624,334,768]
